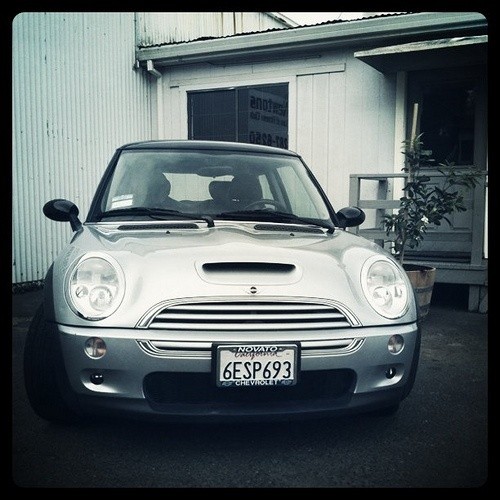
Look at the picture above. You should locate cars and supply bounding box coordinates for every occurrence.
[24,138,422,433]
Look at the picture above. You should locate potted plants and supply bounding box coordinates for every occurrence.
[380,130,491,325]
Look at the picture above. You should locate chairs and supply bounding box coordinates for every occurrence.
[128,170,184,211]
[224,167,265,211]
